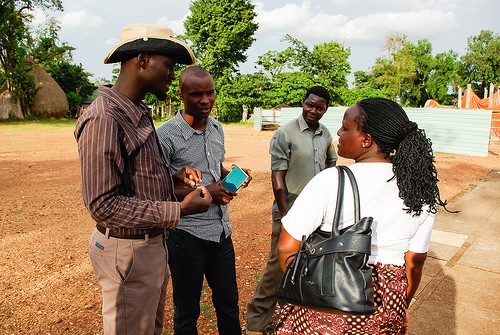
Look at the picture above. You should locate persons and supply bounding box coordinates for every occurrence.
[246,85,337,335]
[156,66,252,335]
[74,25,212,335]
[272,97,461,335]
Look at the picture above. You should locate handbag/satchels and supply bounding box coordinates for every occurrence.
[277,165,375,315]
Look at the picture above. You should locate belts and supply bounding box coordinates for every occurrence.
[96,223,162,239]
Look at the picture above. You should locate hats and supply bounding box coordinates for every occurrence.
[104,24,195,66]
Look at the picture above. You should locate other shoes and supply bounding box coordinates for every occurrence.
[246,324,263,335]
